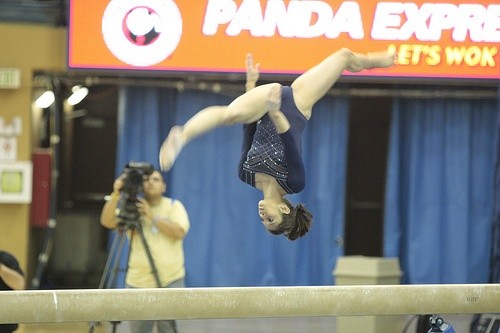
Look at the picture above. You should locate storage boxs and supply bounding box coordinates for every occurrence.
[330,255,406,333]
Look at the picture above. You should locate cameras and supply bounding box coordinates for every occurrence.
[122,162,154,194]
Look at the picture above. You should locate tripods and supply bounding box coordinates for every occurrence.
[89,203,177,333]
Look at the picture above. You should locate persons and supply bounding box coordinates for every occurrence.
[99,163,191,333]
[0,249,26,333]
[156,46,401,240]
